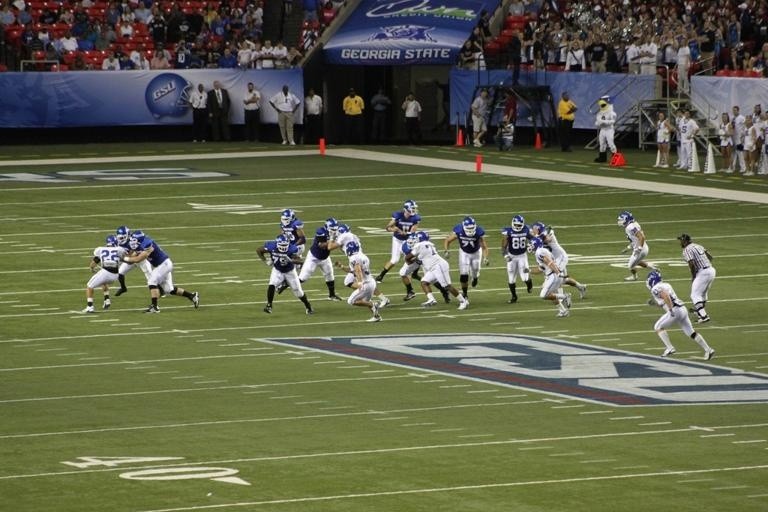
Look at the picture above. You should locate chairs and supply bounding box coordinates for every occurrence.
[296,0,335,57]
[0,0,288,70]
[477,0,768,77]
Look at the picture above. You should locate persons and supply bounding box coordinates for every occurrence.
[445,215,489,299]
[533,222,589,303]
[371,87,391,143]
[457,2,674,76]
[304,86,323,143]
[406,234,470,311]
[717,105,767,177]
[493,114,515,151]
[618,210,655,282]
[270,85,300,145]
[471,87,489,148]
[402,91,422,145]
[677,233,717,324]
[374,197,421,284]
[276,216,343,300]
[594,95,612,161]
[399,236,451,304]
[257,234,314,316]
[647,269,715,360]
[597,100,618,162]
[675,1,767,96]
[114,226,166,299]
[274,209,305,271]
[122,231,199,313]
[503,89,517,123]
[208,81,231,142]
[342,87,365,144]
[558,91,576,151]
[189,83,208,142]
[529,239,572,318]
[335,243,382,324]
[672,108,685,166]
[1,1,348,72]
[679,108,700,169]
[656,111,672,168]
[243,83,261,143]
[328,222,390,310]
[502,213,536,303]
[81,236,128,313]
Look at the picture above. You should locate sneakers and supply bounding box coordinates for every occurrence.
[102,298,111,309]
[403,292,416,301]
[624,275,638,281]
[661,348,676,356]
[192,291,199,308]
[507,297,517,304]
[472,278,478,287]
[689,307,698,314]
[527,286,532,293]
[114,288,127,296]
[282,140,287,145]
[694,317,711,324]
[378,299,390,309]
[375,274,384,281]
[456,300,469,310]
[366,316,383,322]
[444,296,450,304]
[703,349,715,360]
[472,139,482,148]
[463,294,468,300]
[421,299,437,308]
[142,306,161,314]
[81,306,95,312]
[290,142,296,146]
[306,306,314,315]
[411,274,421,281]
[555,311,568,317]
[564,293,571,309]
[654,267,662,277]
[372,302,378,317]
[263,306,273,314]
[578,284,587,297]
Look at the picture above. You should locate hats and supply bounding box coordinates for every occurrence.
[677,234,691,241]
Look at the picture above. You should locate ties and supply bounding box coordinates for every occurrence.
[216,91,222,107]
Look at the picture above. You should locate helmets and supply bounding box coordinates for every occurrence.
[116,226,127,235]
[279,209,295,226]
[338,224,351,236]
[527,239,542,253]
[132,230,144,242]
[463,216,478,238]
[346,240,360,256]
[414,231,430,242]
[403,200,418,216]
[106,235,118,245]
[324,218,338,233]
[617,211,635,228]
[530,222,546,238]
[513,215,522,226]
[646,270,662,290]
[275,234,290,252]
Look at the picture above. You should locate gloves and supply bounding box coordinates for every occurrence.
[503,255,512,262]
[90,268,98,274]
[558,273,569,279]
[670,312,675,318]
[633,247,642,257]
[646,299,655,306]
[621,245,630,254]
[522,267,530,274]
[266,261,274,267]
[483,257,490,266]
[283,255,292,263]
[443,250,450,258]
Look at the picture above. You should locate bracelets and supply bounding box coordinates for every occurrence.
[638,246,643,249]
[358,282,362,285]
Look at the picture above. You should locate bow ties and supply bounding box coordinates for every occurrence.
[327,295,343,301]
